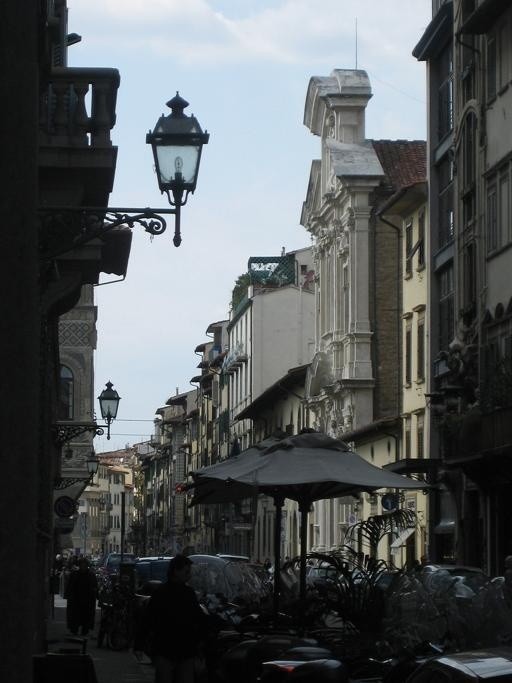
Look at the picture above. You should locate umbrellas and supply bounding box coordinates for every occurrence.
[193,429,302,615]
[188,429,434,636]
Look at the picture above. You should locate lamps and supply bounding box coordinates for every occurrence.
[56,451,100,490]
[55,381,124,443]
[37,91,211,260]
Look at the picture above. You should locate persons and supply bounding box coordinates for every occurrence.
[416,555,426,569]
[51,552,95,600]
[62,557,99,636]
[262,558,273,569]
[132,554,210,683]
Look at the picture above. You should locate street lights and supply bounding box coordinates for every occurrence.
[80,510,87,557]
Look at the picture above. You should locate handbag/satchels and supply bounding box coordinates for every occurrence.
[195,644,207,680]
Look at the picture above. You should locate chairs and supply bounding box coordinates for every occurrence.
[33,637,98,683]
